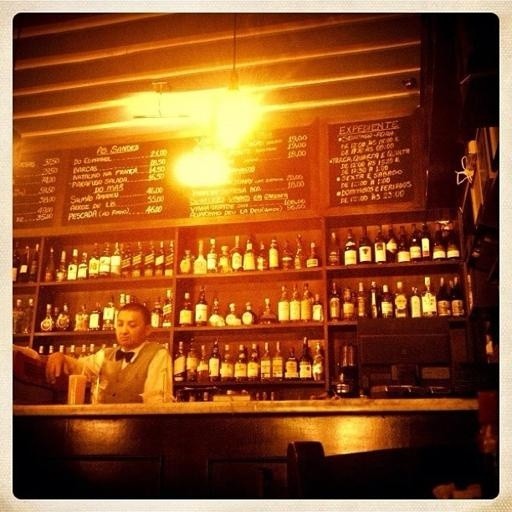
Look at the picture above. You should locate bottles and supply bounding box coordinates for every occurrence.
[14,221,470,401]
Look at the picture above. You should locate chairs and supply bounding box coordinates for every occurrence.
[287,441,499,499]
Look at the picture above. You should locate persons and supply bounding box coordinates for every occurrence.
[44,302,177,404]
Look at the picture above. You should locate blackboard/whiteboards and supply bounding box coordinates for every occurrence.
[322,115,426,214]
[17,143,58,238]
[59,115,318,240]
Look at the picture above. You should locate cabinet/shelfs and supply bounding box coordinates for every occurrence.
[170,209,327,401]
[324,204,472,398]
[13,236,42,348]
[13,219,177,358]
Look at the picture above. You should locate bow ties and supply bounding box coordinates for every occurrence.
[114,349,135,362]
